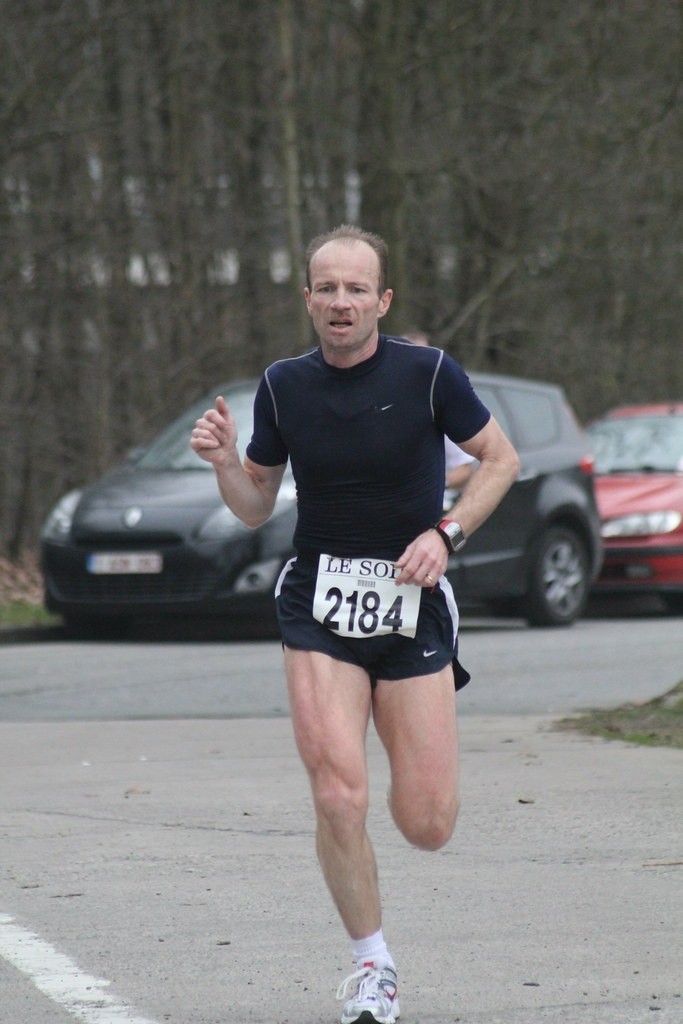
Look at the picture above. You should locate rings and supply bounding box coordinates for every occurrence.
[426,574,436,585]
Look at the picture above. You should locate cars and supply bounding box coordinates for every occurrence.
[39,367,612,629]
[571,395,683,615]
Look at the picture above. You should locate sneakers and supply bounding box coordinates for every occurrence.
[336,956,401,1024]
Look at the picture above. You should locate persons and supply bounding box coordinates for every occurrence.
[190,223,521,1024]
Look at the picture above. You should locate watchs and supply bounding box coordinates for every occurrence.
[431,518,468,555]
